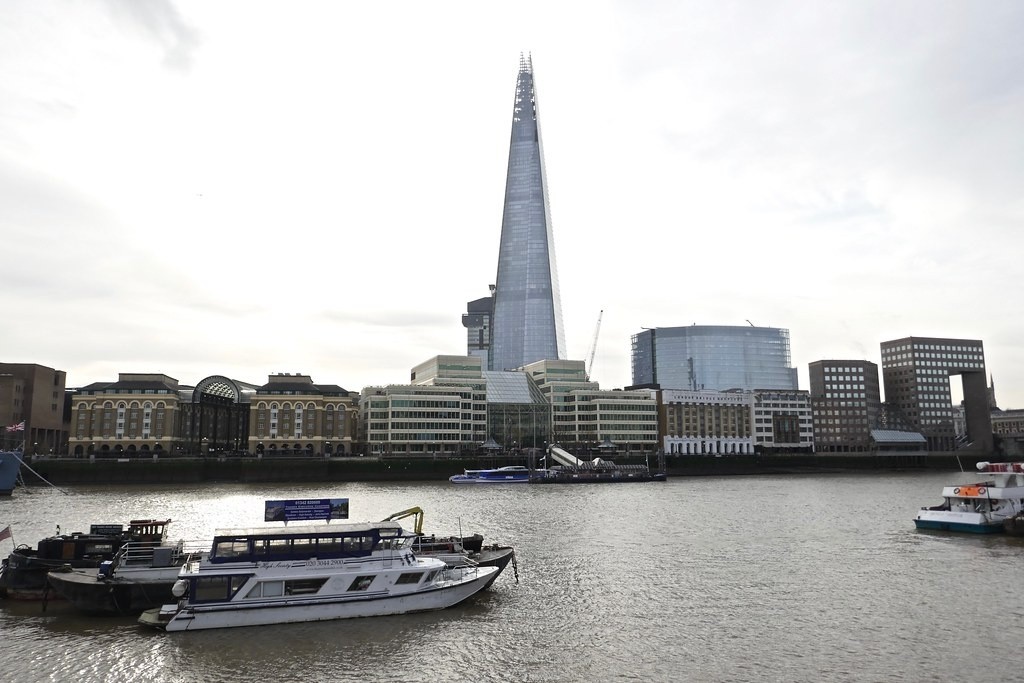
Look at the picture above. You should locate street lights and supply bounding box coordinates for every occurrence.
[514,441,516,454]
[432,442,435,453]
[326,442,329,455]
[34,442,37,453]
[260,441,262,453]
[155,442,158,454]
[91,442,94,455]
[380,442,382,456]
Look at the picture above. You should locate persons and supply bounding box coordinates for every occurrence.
[448,538,454,553]
[429,534,435,543]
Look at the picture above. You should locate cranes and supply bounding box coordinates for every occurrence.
[583,310,602,381]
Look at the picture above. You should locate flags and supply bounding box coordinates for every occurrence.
[6,422,24,431]
[0,527,11,543]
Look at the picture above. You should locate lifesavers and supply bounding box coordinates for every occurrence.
[953,487,961,494]
[977,487,987,495]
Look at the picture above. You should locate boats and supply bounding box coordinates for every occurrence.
[529,457,668,482]
[0,519,201,600]
[449,466,550,484]
[911,461,1024,535]
[138,521,500,633]
[46,506,515,617]
[0,449,23,495]
